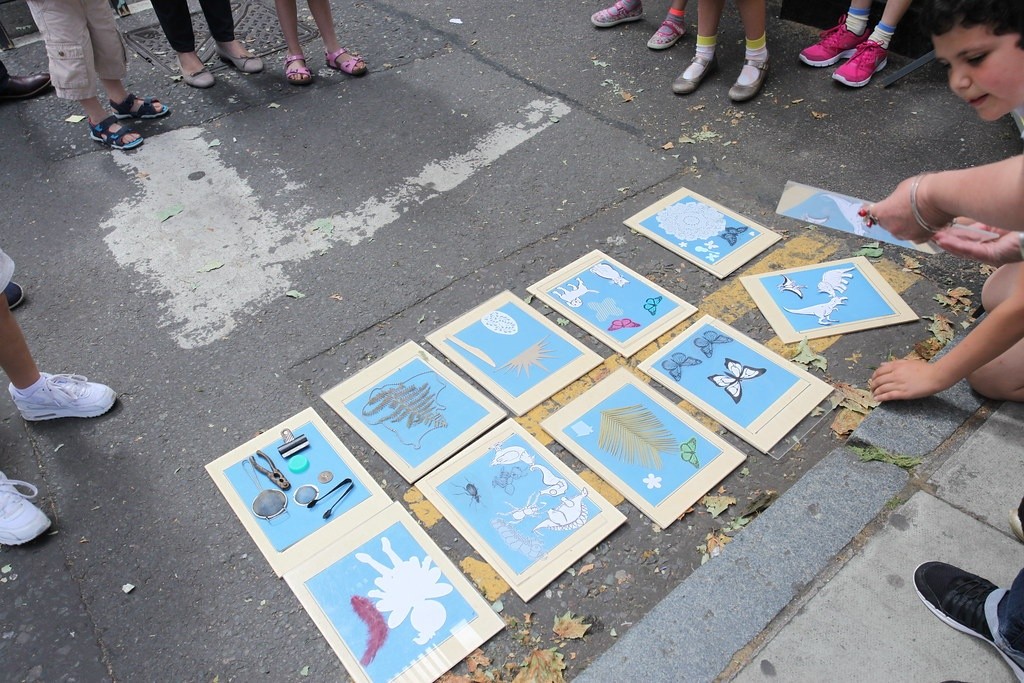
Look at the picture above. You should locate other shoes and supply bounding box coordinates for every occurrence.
[645,21,686,49]
[591,1,644,27]
[4,282,24,310]
[1010,498,1023,541]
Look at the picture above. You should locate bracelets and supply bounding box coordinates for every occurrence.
[909,171,957,234]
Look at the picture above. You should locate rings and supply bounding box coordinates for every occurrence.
[869,215,878,225]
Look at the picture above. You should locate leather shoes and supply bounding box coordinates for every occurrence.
[216,44,262,72]
[176,53,214,89]
[729,59,769,101]
[672,52,717,94]
[2,74,52,99]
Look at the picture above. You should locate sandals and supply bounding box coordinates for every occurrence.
[88,114,143,149]
[325,48,367,76]
[109,94,170,119]
[282,52,312,84]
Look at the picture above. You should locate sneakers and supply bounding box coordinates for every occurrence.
[1,472,52,546]
[912,562,1024,683]
[831,40,888,87]
[798,13,871,67]
[8,372,118,421]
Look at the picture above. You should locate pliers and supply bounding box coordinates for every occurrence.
[249,450,291,490]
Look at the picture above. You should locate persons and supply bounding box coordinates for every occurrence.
[25,0,169,149]
[151,0,264,88]
[274,0,367,84]
[868,152,1024,267]
[869,0,1024,402]
[672,0,771,101]
[912,559,1024,683]
[589,0,689,50]
[0,60,51,100]
[798,0,913,87]
[0,245,117,545]
